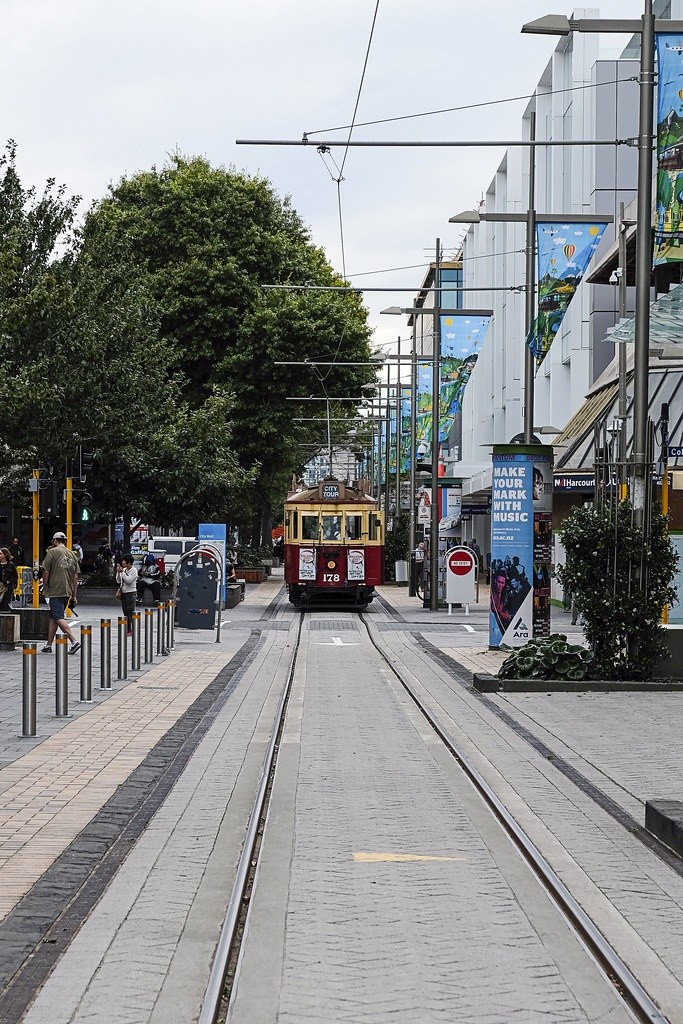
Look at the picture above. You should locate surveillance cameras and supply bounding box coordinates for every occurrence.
[417,444,426,456]
[609,271,618,287]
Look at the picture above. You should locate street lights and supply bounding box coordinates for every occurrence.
[448,207,564,443]
[360,383,419,528]
[516,0,683,589]
[367,351,442,597]
[380,304,494,612]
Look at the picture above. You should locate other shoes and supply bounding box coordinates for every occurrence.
[127,631,132,636]
[153,600,159,604]
[136,598,142,602]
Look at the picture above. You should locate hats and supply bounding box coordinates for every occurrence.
[53,532,66,538]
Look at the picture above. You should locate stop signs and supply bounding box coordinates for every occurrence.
[447,552,473,575]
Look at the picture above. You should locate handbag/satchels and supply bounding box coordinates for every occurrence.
[116,589,122,599]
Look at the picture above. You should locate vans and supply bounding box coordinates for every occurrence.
[146,535,199,578]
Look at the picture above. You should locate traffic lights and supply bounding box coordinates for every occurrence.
[79,437,101,478]
[78,490,93,524]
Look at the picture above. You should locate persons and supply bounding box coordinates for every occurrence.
[226,558,236,583]
[6,535,25,565]
[533,467,543,500]
[273,536,284,565]
[72,537,83,563]
[41,532,81,655]
[547,522,551,533]
[536,564,546,579]
[472,539,480,555]
[0,548,18,611]
[227,549,238,567]
[415,542,425,591]
[324,517,348,540]
[97,538,123,584]
[460,541,468,550]
[571,598,578,625]
[467,542,478,581]
[135,555,161,603]
[116,554,138,635]
[492,556,524,622]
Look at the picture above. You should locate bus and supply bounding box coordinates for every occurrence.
[282,400,387,611]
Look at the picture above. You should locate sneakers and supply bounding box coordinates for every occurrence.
[41,646,52,653]
[68,642,81,654]
